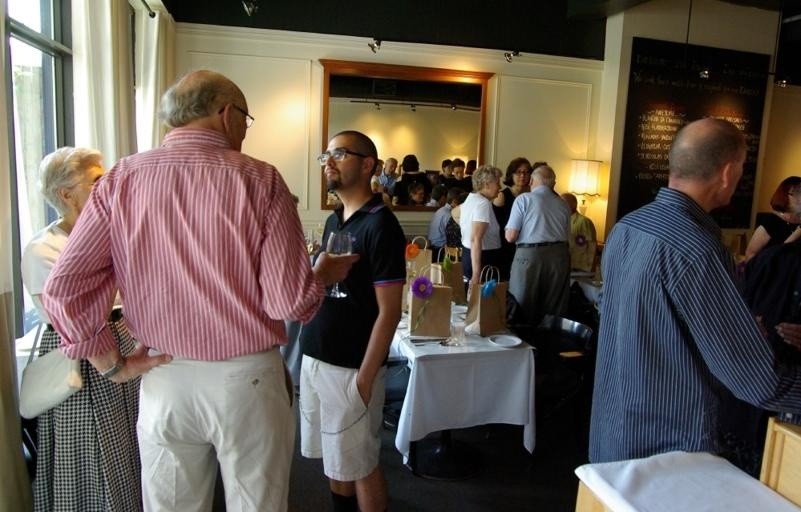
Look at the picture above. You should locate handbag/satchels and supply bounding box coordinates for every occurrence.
[465,265,508,338]
[19,347,83,419]
[400,236,466,342]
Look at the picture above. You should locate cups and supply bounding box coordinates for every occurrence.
[450,320,466,346]
[302,229,315,256]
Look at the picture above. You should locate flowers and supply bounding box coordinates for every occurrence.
[405,242,422,260]
[443,255,455,273]
[482,279,497,300]
[412,275,433,300]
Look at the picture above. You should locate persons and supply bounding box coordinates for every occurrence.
[370,153,476,206]
[586,114,801,464]
[298,129,408,511]
[42,68,362,511]
[742,245,801,350]
[19,146,144,511]
[745,176,801,261]
[429,156,598,322]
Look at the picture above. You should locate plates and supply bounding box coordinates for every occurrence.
[591,281,603,285]
[452,305,468,314]
[487,335,523,348]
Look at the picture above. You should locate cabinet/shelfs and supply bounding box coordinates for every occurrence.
[758,401,800,507]
[573,448,800,512]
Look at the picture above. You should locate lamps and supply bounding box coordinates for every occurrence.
[503,48,524,61]
[368,37,383,52]
[565,154,605,216]
[371,102,462,114]
[767,68,791,88]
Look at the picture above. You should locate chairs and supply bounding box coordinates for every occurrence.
[524,307,597,402]
[284,309,416,478]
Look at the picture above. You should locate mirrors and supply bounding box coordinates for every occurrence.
[314,54,499,215]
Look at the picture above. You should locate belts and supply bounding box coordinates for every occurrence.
[518,242,563,247]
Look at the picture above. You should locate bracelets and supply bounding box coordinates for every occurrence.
[99,353,127,379]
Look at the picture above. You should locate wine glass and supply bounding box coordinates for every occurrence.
[325,231,353,301]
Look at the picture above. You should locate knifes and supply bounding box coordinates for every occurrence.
[410,338,446,343]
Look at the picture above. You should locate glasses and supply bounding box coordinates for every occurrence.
[317,148,367,165]
[219,103,254,128]
